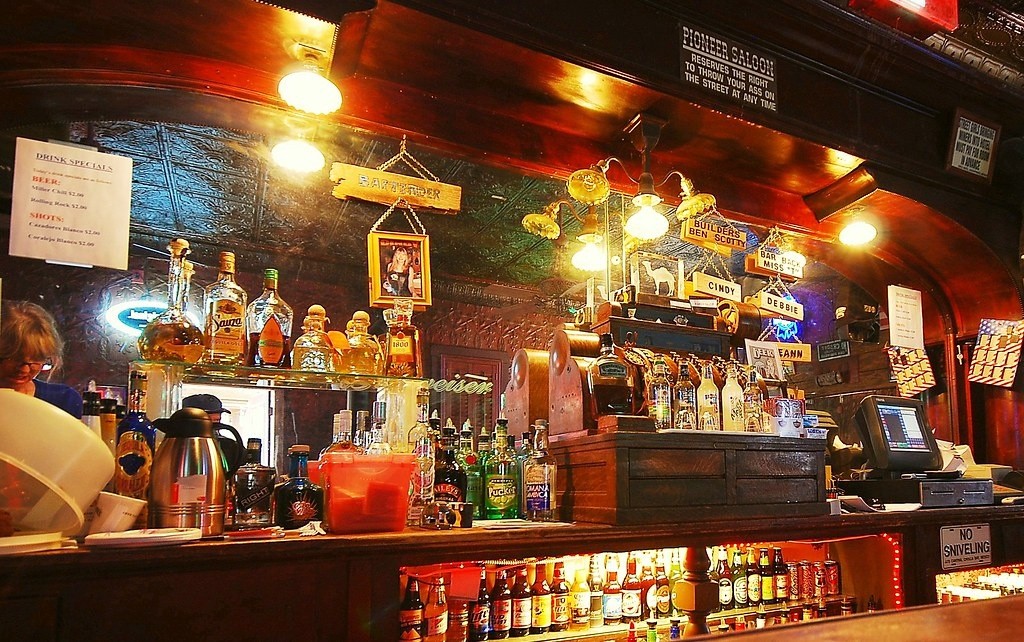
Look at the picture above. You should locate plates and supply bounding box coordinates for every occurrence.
[84,529,202,549]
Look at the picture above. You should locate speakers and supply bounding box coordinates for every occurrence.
[835,281,880,345]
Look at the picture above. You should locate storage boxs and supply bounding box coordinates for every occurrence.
[319,453,416,535]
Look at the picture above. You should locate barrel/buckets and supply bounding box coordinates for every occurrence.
[319,454,418,534]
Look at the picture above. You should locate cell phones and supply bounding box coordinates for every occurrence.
[901,474,927,479]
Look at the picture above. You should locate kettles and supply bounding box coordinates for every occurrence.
[149,394,244,542]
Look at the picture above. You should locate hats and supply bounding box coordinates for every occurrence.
[183,394,231,414]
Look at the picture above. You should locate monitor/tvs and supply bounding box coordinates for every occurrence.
[851,395,943,479]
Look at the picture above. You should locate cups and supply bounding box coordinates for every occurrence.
[88,491,149,535]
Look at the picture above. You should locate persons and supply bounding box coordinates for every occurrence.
[0,299,83,421]
[183,394,235,452]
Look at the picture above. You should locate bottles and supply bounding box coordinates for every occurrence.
[133,238,205,365]
[673,364,697,430]
[232,439,275,531]
[246,269,293,368]
[626,595,877,642]
[405,389,435,525]
[291,299,423,379]
[202,249,248,366]
[275,445,324,531]
[722,364,744,431]
[707,547,787,613]
[468,547,684,642]
[325,401,394,454]
[400,576,448,642]
[647,360,672,430]
[935,568,1023,605]
[586,333,634,422]
[697,367,721,431]
[744,366,765,432]
[80,369,156,531]
[429,409,559,529]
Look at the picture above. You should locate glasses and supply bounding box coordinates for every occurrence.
[2,356,53,373]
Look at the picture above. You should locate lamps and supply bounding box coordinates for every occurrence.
[271,114,327,171]
[566,117,717,241]
[522,188,655,275]
[840,204,878,245]
[278,44,341,114]
